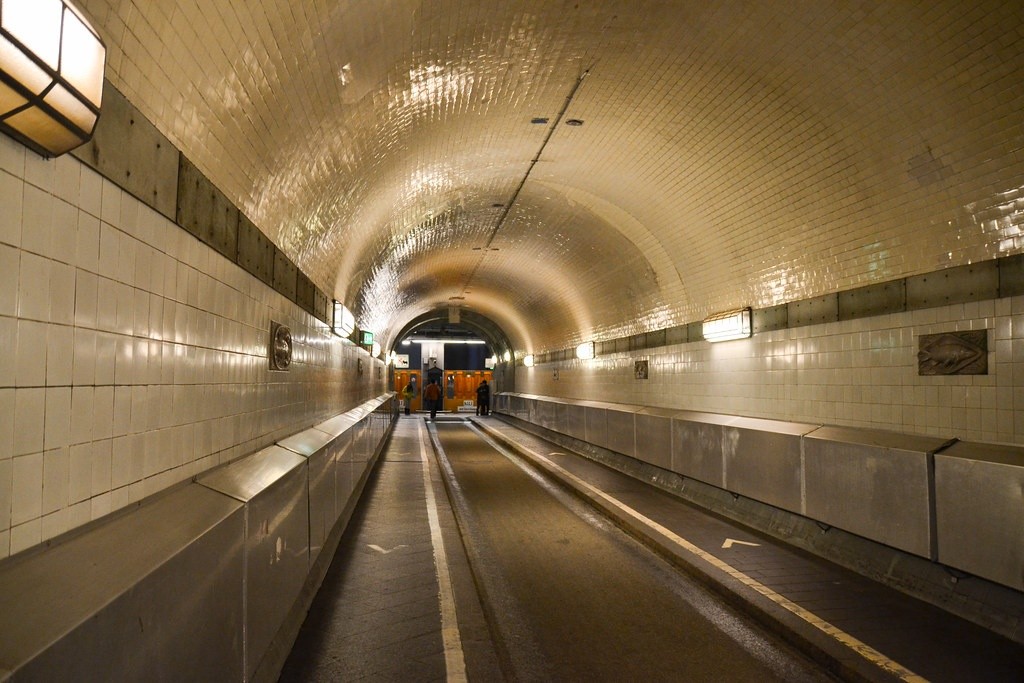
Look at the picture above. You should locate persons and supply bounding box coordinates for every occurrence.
[402,381,414,415]
[475,381,490,416]
[425,378,441,420]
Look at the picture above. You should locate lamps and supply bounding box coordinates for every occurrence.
[332,299,355,339]
[0,0,107,159]
[702,306,752,344]
